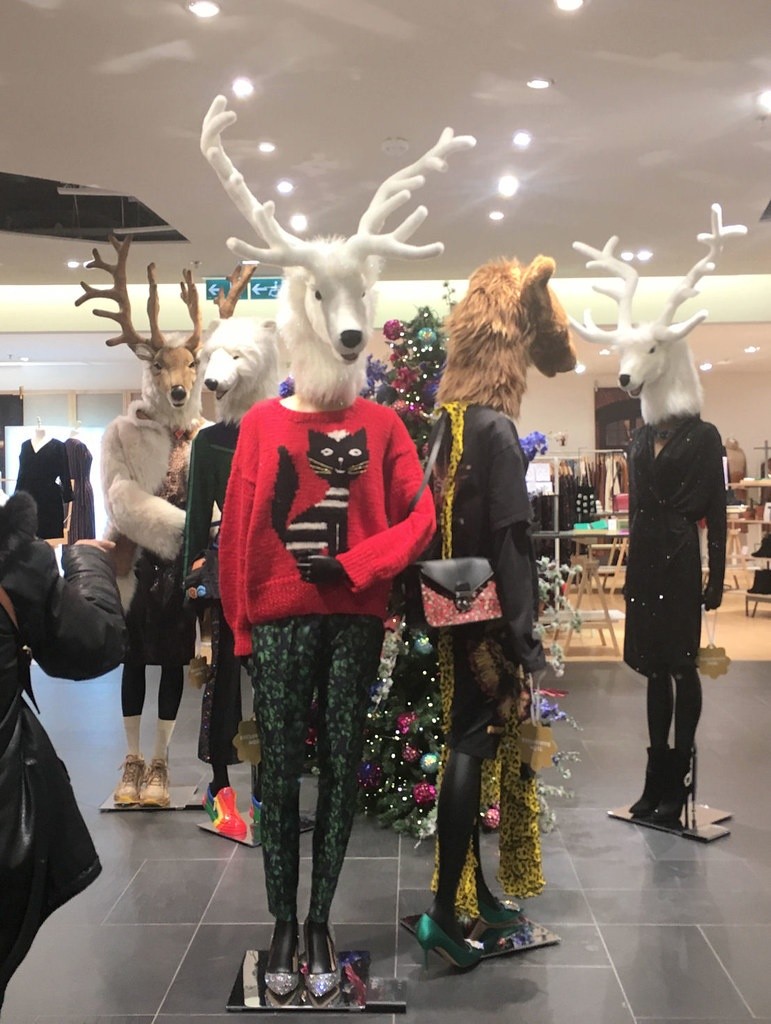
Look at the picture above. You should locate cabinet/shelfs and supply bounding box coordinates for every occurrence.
[726,483,771,525]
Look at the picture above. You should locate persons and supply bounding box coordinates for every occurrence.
[181,320,278,835]
[219,251,436,999]
[418,256,576,967]
[0,486,128,1010]
[615,325,726,822]
[101,342,222,806]
[9,428,97,571]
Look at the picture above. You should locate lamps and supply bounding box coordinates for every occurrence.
[114,196,176,234]
[57,183,133,196]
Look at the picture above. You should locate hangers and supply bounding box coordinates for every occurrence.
[550,451,624,478]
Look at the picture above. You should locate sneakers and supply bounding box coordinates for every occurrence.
[248,794,262,823]
[201,782,247,841]
[139,759,171,808]
[112,753,145,805]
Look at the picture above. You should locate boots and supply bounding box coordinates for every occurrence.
[628,743,695,824]
[747,569,771,595]
[751,533,771,558]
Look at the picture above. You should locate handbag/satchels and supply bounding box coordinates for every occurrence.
[575,463,597,515]
[610,466,629,512]
[180,547,221,610]
[417,556,503,629]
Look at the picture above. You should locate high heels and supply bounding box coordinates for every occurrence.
[264,916,300,996]
[477,899,525,926]
[414,912,485,970]
[303,915,341,998]
